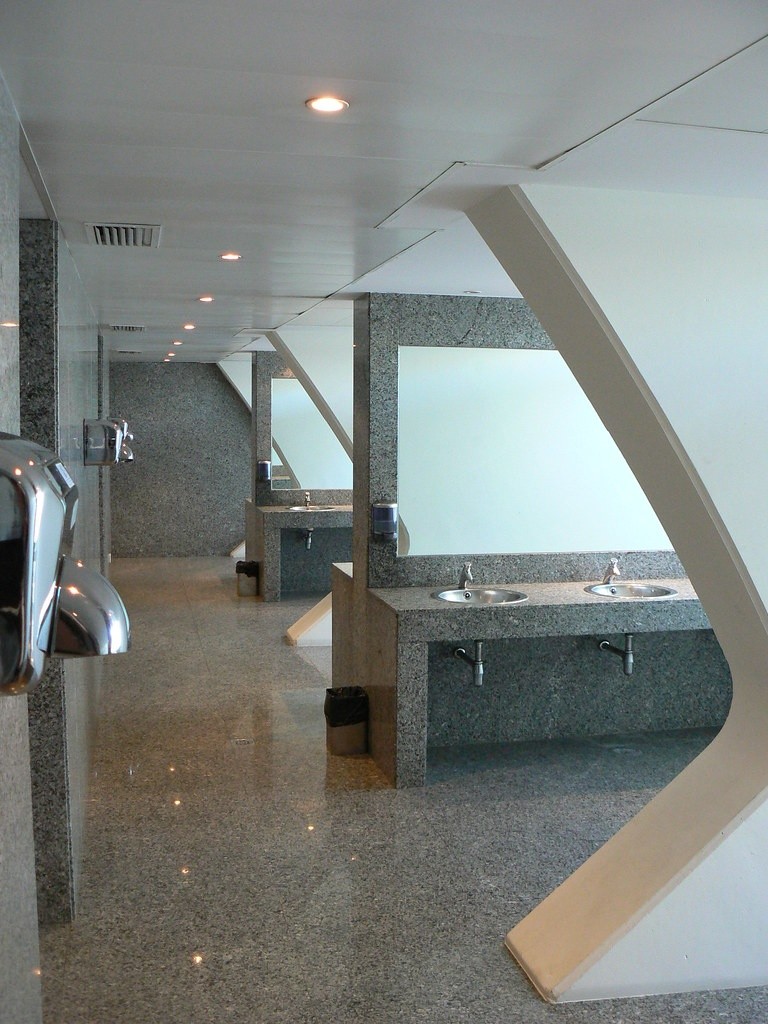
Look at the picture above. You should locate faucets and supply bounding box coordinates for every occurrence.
[305,492,310,506]
[602,558,622,585]
[458,561,474,588]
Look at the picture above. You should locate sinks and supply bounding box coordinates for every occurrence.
[285,505,337,511]
[430,587,529,606]
[584,583,679,599]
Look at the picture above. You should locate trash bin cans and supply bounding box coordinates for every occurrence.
[236,559,258,597]
[323,685,367,757]
